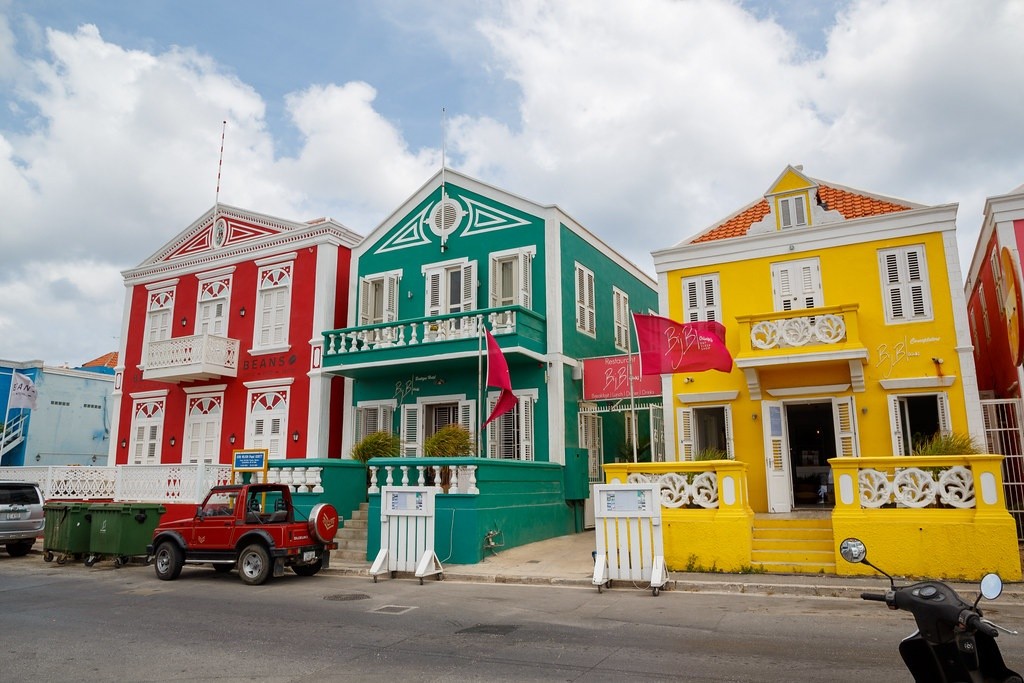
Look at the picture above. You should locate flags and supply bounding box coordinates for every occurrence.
[629,310,733,382]
[10,373,38,410]
[481,326,517,430]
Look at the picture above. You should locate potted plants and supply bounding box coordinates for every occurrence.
[425,423,478,495]
[910,431,986,508]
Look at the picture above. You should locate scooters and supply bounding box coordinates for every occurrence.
[840,536,1024,683]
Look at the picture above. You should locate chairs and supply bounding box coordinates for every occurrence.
[245,511,263,524]
[268,509,289,524]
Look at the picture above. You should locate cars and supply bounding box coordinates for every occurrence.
[0,478,48,552]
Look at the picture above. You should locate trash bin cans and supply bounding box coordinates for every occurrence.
[88,502,167,566]
[41,500,91,564]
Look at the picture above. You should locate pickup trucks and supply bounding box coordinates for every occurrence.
[146,482,340,587]
